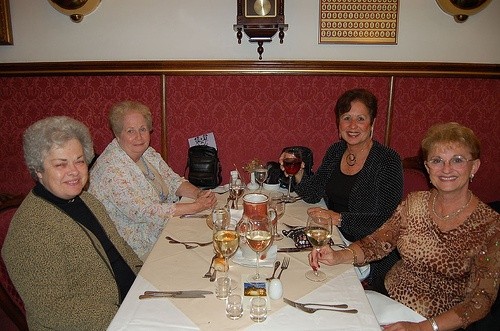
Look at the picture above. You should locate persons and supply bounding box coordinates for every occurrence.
[85,100,219,257]
[308,122,500,331]
[1,115,144,331]
[278,88,405,299]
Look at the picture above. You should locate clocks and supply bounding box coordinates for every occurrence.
[233,0,288,60]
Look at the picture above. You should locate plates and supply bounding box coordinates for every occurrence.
[206,209,243,230]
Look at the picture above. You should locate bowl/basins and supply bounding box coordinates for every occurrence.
[262,182,280,192]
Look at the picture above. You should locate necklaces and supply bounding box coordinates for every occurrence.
[134,155,167,203]
[344,139,372,166]
[432,189,473,221]
[66,199,75,203]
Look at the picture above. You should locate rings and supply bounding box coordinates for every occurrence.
[319,210,322,214]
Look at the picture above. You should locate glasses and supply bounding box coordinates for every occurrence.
[424,155,472,169]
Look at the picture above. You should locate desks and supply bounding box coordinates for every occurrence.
[107,186,382,331]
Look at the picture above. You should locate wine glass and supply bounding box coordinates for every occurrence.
[212,217,239,290]
[254,161,268,192]
[306,210,332,282]
[229,176,245,210]
[281,149,302,203]
[269,191,285,241]
[244,214,272,283]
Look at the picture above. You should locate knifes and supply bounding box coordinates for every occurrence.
[210,270,216,282]
[144,290,214,294]
[139,294,206,299]
[277,247,312,252]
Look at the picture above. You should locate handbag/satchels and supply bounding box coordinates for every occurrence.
[280,146,313,193]
[184,144,223,190]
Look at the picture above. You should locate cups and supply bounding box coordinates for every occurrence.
[242,193,278,231]
[250,297,267,322]
[215,271,232,301]
[212,204,231,231]
[225,294,244,320]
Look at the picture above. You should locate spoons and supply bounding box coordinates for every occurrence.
[265,261,280,281]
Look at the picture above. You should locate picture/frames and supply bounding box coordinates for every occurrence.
[318,0,400,45]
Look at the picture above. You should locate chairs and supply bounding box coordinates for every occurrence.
[0,192,29,331]
[402,156,434,197]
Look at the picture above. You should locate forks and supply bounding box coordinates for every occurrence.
[212,190,230,195]
[168,241,213,247]
[283,297,348,308]
[203,254,217,278]
[277,257,290,279]
[165,236,198,249]
[294,304,358,314]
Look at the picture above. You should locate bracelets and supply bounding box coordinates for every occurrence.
[427,316,439,331]
[338,213,342,227]
[343,247,357,266]
[197,189,203,198]
